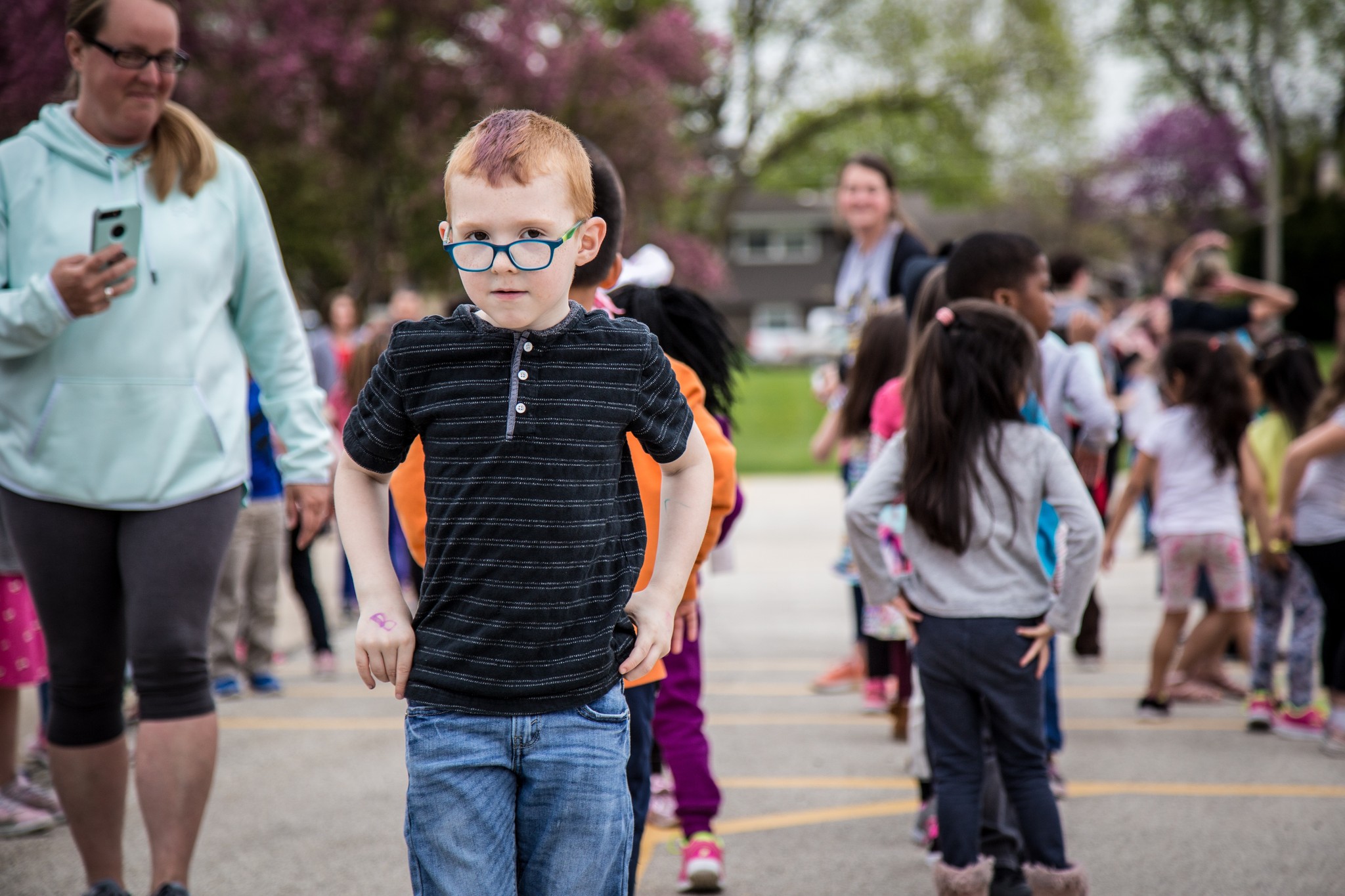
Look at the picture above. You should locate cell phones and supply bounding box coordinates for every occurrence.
[88,203,144,299]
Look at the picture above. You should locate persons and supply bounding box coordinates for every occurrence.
[804,162,1118,896]
[391,145,746,896]
[0,1,332,896]
[1105,230,1345,751]
[0,491,139,837]
[341,107,715,896]
[208,271,402,703]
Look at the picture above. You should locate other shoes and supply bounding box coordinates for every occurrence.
[675,831,728,896]
[0,774,67,823]
[0,792,54,839]
[1245,689,1278,731]
[19,747,55,788]
[860,672,899,716]
[1273,701,1326,743]
[810,655,866,693]
[213,673,238,697]
[246,670,280,693]
[646,774,683,828]
[1135,696,1168,718]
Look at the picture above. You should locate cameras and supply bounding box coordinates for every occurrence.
[1191,248,1227,287]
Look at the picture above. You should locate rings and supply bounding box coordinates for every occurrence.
[104,287,112,301]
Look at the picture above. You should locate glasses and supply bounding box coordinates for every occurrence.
[442,217,583,273]
[82,29,188,75]
